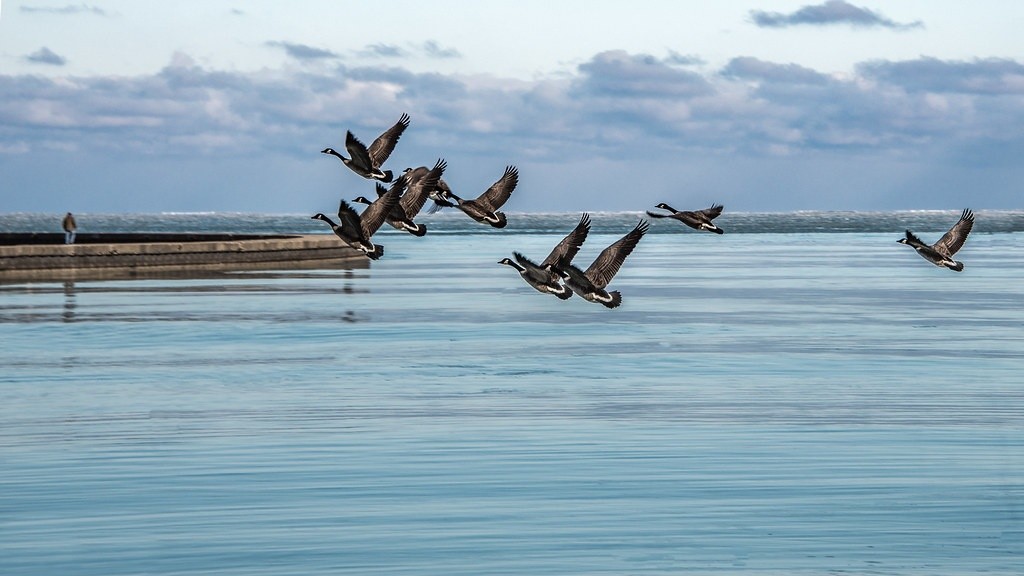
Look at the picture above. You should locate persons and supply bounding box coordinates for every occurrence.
[62,211,77,243]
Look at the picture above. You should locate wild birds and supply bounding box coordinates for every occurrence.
[646,203,724,235]
[310,175,413,261]
[497,212,591,300]
[390,168,451,214]
[320,111,411,184]
[895,208,975,271]
[352,157,447,237]
[541,218,650,309]
[433,165,519,229]
